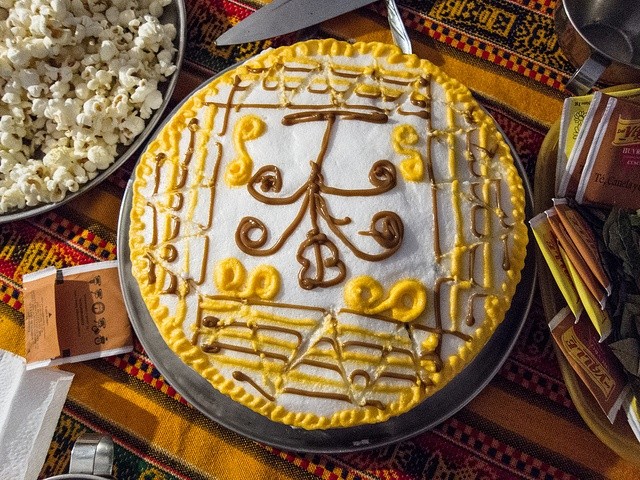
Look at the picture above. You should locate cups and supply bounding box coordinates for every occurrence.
[41,429,125,480]
[553,0,639,95]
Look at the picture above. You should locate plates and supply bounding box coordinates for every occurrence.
[0,0,187,224]
[534,85,640,469]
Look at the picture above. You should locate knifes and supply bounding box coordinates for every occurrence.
[213,0,379,46]
